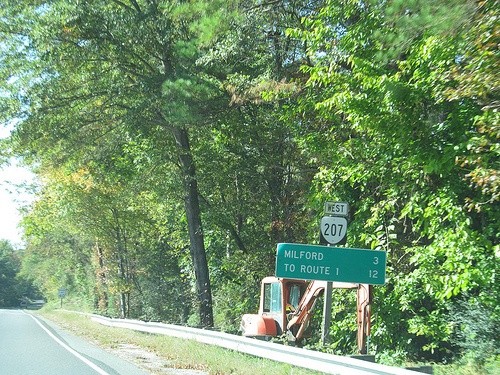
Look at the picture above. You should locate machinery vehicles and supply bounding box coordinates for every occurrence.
[239,276,376,363]
